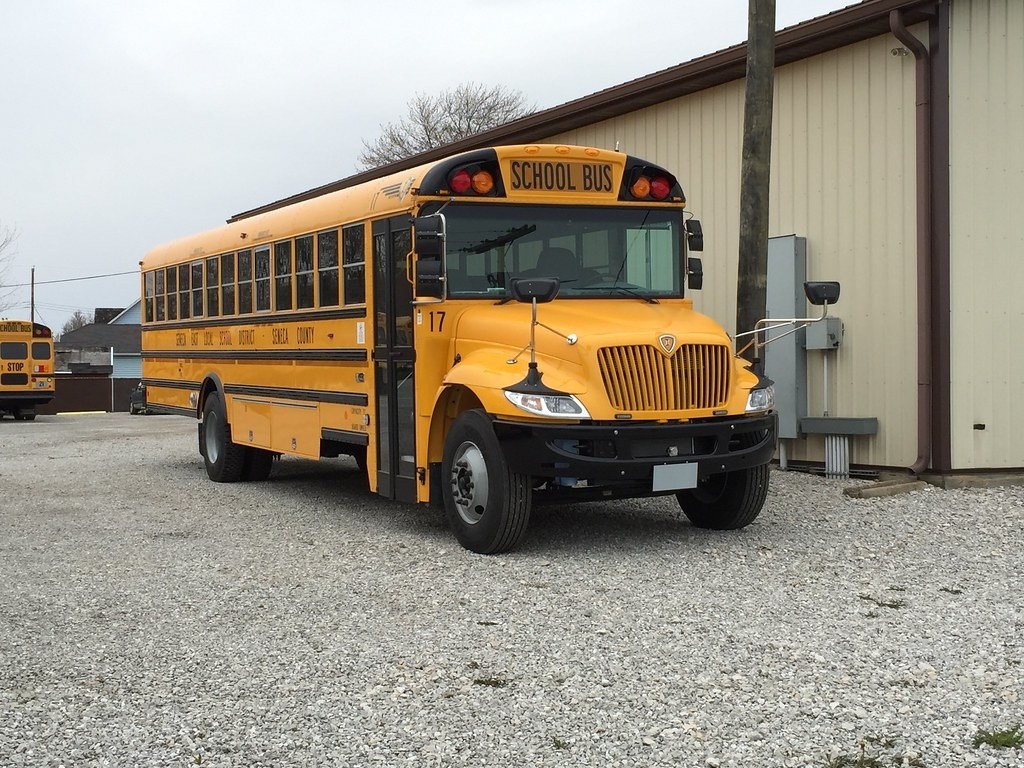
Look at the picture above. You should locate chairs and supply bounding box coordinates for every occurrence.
[538,247,580,283]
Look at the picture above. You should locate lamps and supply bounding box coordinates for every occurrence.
[891,48,908,56]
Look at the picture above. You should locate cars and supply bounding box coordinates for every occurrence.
[129,382,153,415]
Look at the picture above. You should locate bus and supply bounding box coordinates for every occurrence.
[0,317,56,421]
[138,140,842,555]
